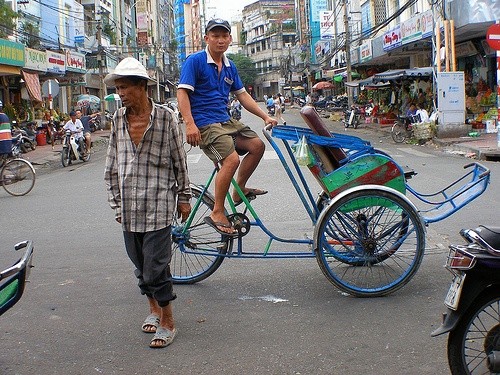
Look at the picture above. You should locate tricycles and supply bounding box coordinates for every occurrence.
[161,119,489,298]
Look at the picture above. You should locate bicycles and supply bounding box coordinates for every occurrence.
[0,150,36,197]
[391,115,419,143]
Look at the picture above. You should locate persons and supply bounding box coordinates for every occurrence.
[400,89,429,124]
[305,92,312,106]
[75,109,102,155]
[167,99,183,124]
[229,98,241,116]
[103,56,193,348]
[267,93,287,125]
[0,101,12,155]
[318,94,324,101]
[63,111,85,154]
[80,103,91,115]
[176,19,278,237]
[16,108,60,145]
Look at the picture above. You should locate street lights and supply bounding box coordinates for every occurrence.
[155,33,189,104]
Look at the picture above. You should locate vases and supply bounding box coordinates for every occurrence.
[37,133,46,146]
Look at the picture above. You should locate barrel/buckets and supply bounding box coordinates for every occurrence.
[36,134,46,145]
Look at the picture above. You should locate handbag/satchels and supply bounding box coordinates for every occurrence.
[295,135,311,166]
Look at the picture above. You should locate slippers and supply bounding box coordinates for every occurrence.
[142,315,160,333]
[203,216,239,236]
[234,189,268,203]
[149,326,178,348]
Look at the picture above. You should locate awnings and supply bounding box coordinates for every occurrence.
[358,67,444,87]
[325,67,364,83]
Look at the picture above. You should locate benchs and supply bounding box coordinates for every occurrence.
[302,106,352,169]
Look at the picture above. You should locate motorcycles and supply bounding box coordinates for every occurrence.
[57,128,94,167]
[266,102,285,116]
[343,109,360,129]
[429,222,500,375]
[325,100,349,113]
[231,105,241,120]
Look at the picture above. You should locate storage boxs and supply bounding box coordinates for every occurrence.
[472,105,497,134]
[376,112,398,126]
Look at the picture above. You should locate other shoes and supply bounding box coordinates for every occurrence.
[283,121,286,126]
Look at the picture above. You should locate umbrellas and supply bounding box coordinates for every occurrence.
[104,93,121,110]
[345,75,391,106]
[312,81,336,96]
[77,94,101,106]
[293,86,305,95]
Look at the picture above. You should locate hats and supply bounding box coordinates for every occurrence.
[104,57,157,86]
[206,18,231,34]
[70,111,76,116]
